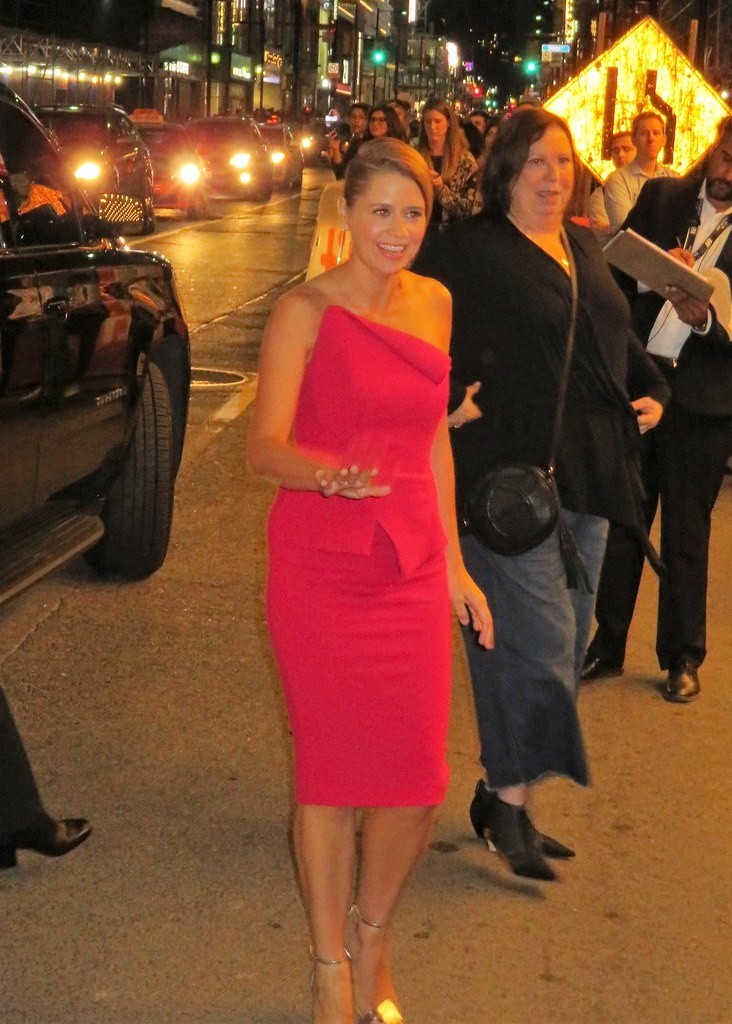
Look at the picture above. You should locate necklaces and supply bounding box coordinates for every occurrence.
[510,213,570,267]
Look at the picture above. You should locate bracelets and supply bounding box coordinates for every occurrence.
[692,322,706,332]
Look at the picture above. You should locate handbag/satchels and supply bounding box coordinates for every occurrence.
[461,461,557,558]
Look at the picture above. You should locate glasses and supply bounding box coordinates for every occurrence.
[370,116,389,122]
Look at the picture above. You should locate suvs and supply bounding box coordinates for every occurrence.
[0,81,190,598]
[179,109,274,203]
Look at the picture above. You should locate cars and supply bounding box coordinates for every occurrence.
[284,119,321,165]
[127,109,209,219]
[32,102,155,235]
[255,117,305,192]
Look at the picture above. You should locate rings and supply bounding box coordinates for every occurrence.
[454,423,462,428]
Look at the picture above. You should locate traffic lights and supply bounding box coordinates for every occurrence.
[373,53,383,63]
[473,87,481,96]
[526,62,537,73]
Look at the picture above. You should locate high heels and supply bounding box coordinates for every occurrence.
[469,784,556,881]
[347,905,404,1024]
[309,944,353,1024]
[0,818,92,869]
[474,778,576,859]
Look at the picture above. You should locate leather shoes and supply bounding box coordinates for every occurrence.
[580,653,624,679]
[662,657,702,702]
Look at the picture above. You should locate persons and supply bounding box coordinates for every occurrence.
[565,163,610,239]
[577,114,732,703]
[325,96,543,241]
[602,112,680,246]
[428,106,671,881]
[247,137,494,1024]
[590,132,637,222]
[254,108,273,123]
[0,681,94,870]
